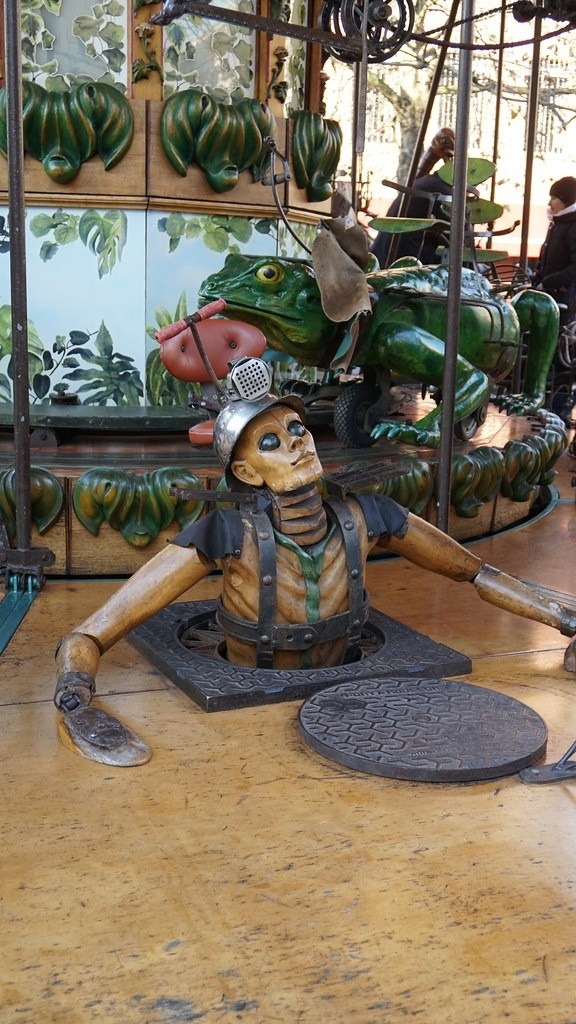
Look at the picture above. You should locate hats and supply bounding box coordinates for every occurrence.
[550,176,576,208]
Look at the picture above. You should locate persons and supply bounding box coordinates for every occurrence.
[514,177,576,443]
[50,369,576,754]
[370,160,488,276]
